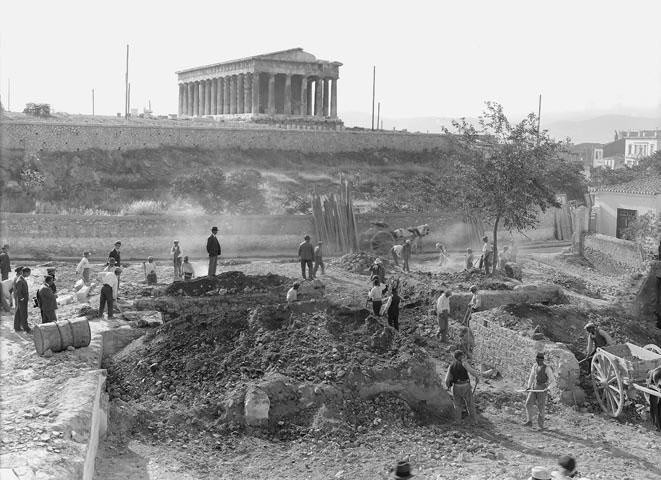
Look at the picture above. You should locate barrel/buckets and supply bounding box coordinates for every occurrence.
[34,317,91,353]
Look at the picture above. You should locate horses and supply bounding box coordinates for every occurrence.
[650,368,661,431]
[393,224,429,254]
[389,239,412,272]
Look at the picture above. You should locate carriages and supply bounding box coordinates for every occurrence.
[361,220,430,259]
[590,342,660,436]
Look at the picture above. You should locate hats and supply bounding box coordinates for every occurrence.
[374,258,383,265]
[83,239,189,274]
[211,227,218,231]
[584,323,596,330]
[394,460,415,480]
[560,455,576,471]
[47,266,56,274]
[533,352,546,362]
[533,466,552,480]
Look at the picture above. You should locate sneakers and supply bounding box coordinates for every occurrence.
[523,421,533,427]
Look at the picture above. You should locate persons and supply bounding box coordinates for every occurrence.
[0,239,196,334]
[313,240,325,277]
[551,456,579,480]
[206,226,221,276]
[585,323,616,384]
[435,292,453,342]
[369,240,415,327]
[445,350,481,425]
[527,466,551,480]
[286,282,301,304]
[460,286,483,326]
[297,235,314,281]
[521,354,553,430]
[435,242,450,266]
[466,234,518,277]
[391,462,413,480]
